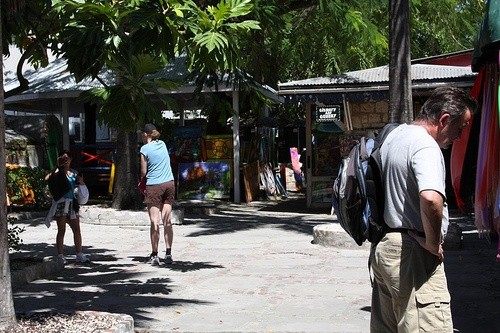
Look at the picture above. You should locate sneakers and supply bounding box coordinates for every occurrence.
[58,254,68,265]
[76,253,90,263]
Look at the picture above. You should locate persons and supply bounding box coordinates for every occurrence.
[137,123,176,265]
[44,151,91,263]
[370,85,476,333]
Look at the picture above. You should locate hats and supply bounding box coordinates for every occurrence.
[141,123,156,136]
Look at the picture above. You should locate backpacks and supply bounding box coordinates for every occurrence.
[332,122,400,246]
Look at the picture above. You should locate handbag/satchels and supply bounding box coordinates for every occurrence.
[77,184,89,204]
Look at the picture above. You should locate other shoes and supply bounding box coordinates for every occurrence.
[144,255,159,266]
[164,254,173,268]
